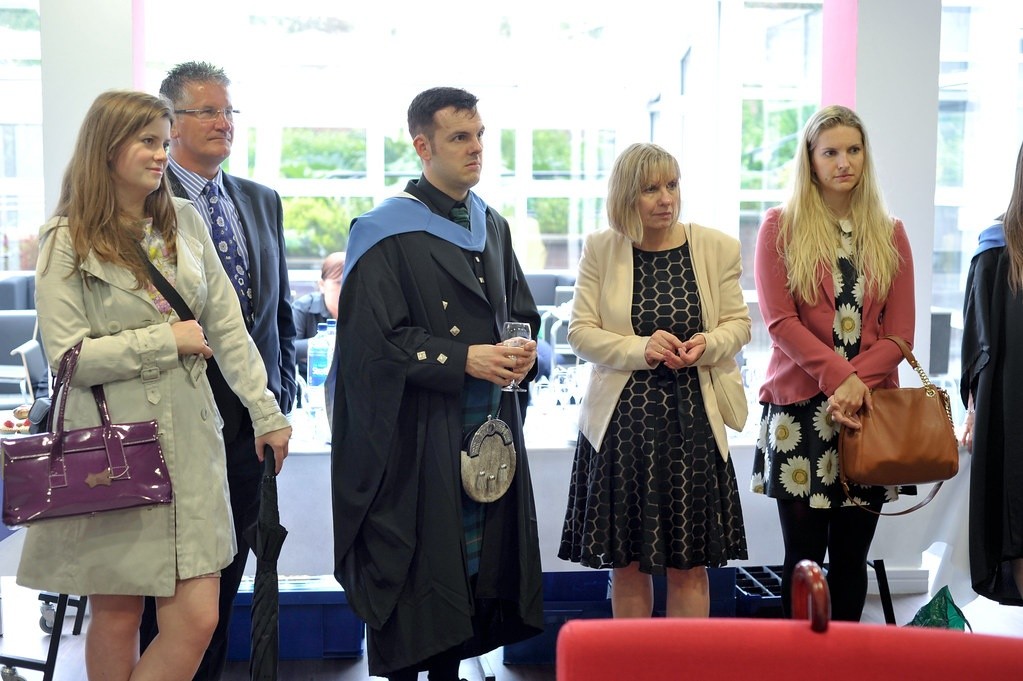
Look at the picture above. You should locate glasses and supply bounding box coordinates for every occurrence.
[173,107,242,122]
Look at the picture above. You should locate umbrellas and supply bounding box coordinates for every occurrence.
[242,443,288,681]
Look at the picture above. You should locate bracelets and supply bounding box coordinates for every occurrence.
[966,410,975,414]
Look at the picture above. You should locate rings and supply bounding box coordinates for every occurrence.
[661,347,665,352]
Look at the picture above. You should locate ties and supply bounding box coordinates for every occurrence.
[447,202,471,235]
[202,180,254,331]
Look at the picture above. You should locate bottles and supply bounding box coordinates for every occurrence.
[327,318,336,369]
[306,322,332,409]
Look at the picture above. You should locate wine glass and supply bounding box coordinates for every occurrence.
[501,322,532,392]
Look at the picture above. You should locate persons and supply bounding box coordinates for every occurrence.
[751,106,916,621]
[15,91,291,681]
[331,86,545,681]
[140,61,297,681]
[556,140,751,619]
[959,141,1023,608]
[292,252,348,382]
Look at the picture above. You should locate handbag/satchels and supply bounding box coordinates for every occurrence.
[837,335,960,516]
[0,341,174,526]
[902,585,974,632]
[460,413,518,505]
